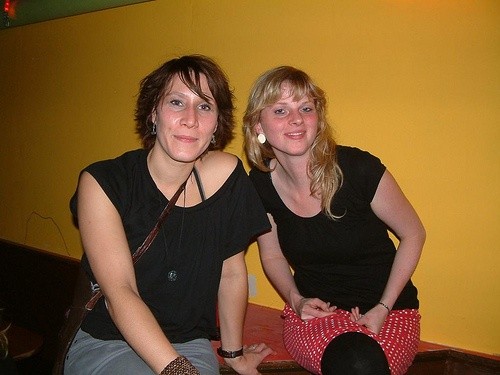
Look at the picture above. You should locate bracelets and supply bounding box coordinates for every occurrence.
[159,355,200,375]
[216,347,243,359]
[380,302,391,313]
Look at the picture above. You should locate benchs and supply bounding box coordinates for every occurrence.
[1,239,500,374]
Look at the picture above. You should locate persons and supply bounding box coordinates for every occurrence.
[64,55,274,375]
[242,65,426,375]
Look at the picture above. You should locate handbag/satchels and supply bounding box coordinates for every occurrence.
[54,263,95,375]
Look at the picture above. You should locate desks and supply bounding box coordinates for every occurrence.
[1,322,45,359]
